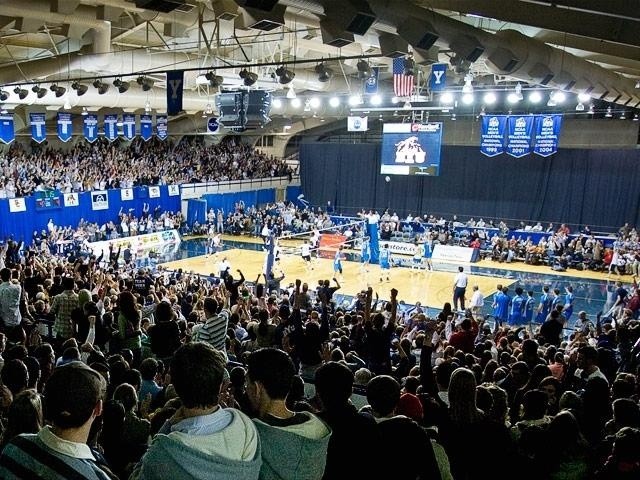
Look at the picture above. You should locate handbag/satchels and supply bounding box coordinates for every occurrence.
[124,322,136,338]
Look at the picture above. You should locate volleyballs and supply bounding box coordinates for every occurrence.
[385,176,390,182]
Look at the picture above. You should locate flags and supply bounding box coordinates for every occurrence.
[392,58,416,98]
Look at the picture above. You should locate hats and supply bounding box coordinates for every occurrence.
[398,393,425,417]
[156,302,172,321]
[78,289,92,302]
[44,362,106,426]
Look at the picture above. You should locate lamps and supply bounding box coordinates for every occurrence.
[0,0,640,121]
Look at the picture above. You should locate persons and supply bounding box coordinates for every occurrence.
[348,266,640,480]
[260,218,281,281]
[611,281,640,319]
[1,235,158,479]
[0,132,293,201]
[32,207,183,254]
[333,246,347,277]
[149,268,379,479]
[302,228,322,270]
[344,209,640,283]
[217,256,230,277]
[194,202,333,255]
